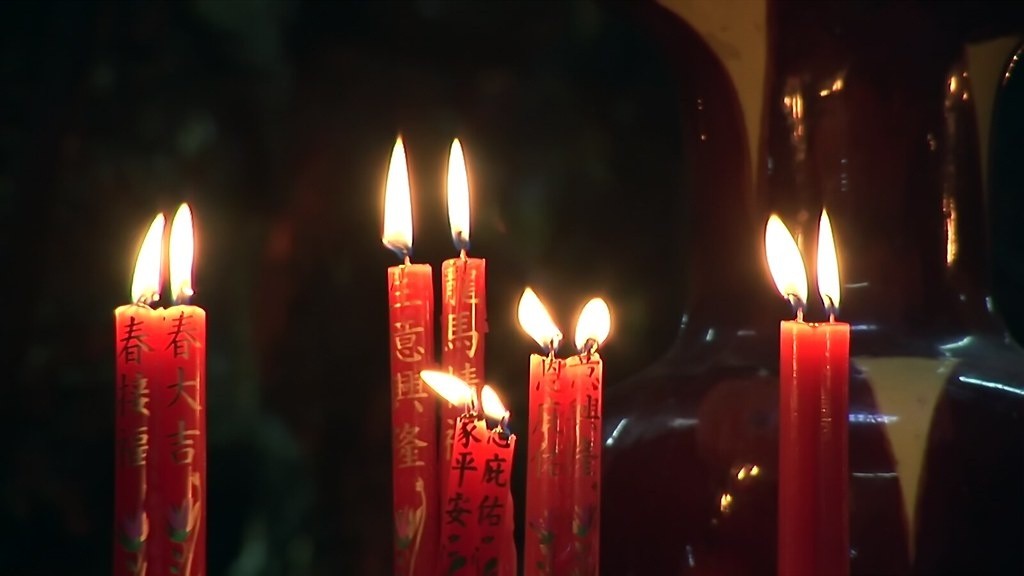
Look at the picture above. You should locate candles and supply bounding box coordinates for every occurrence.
[762,210,853,576]
[380,133,609,576]
[114,203,211,576]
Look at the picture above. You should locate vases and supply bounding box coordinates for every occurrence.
[604,0,1024,576]
